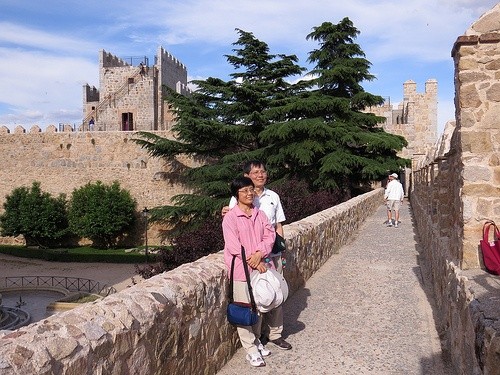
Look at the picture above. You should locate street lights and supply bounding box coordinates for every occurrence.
[143,207,149,264]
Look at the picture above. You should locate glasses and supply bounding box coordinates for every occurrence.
[247,170,267,175]
[238,189,254,194]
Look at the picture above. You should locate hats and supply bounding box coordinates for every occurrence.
[390,173,398,178]
[245,267,288,313]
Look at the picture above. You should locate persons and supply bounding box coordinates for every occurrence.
[89,115,94,131]
[222,177,276,367]
[222,160,293,350]
[385,174,404,205]
[383,173,403,227]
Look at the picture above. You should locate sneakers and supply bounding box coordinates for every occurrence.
[395,223,398,227]
[254,339,271,358]
[246,352,266,367]
[387,221,392,227]
[264,336,291,349]
[259,334,267,344]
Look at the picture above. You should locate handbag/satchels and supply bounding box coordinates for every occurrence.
[271,232,286,254]
[227,302,258,326]
[480,220,500,275]
